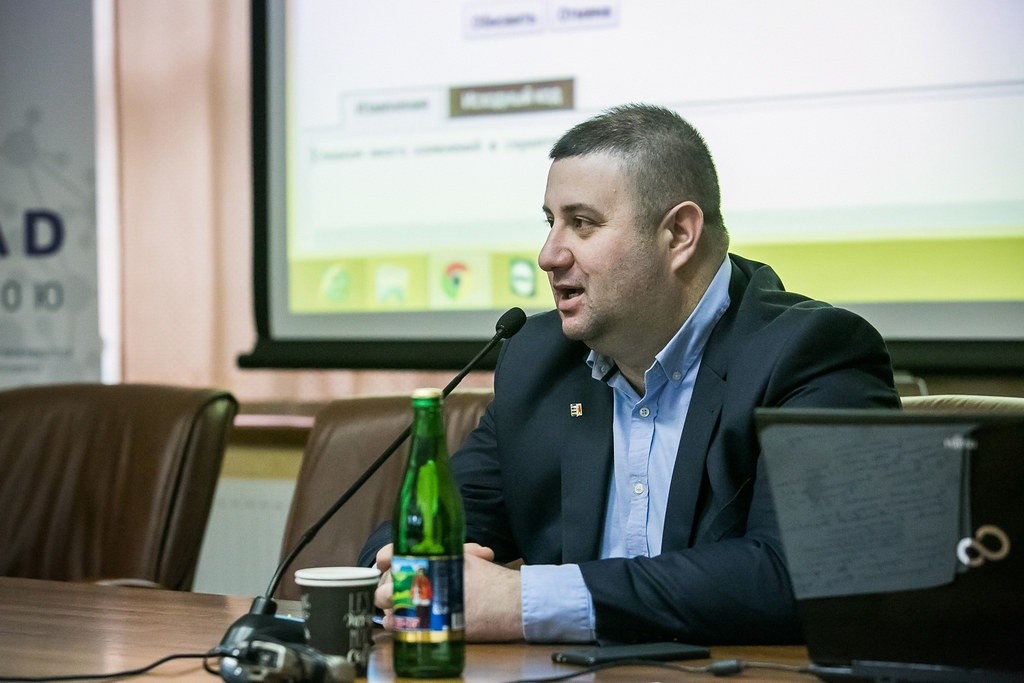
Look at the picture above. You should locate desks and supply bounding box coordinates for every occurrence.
[0,574,820,683]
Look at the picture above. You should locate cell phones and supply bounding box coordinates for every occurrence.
[552,642,711,666]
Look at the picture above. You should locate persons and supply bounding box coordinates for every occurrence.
[352,101,902,644]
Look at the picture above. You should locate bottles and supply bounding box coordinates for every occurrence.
[390,387,466,680]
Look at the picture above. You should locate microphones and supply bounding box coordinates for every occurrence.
[220,307,527,651]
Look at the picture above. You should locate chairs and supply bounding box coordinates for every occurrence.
[275,392,496,601]
[0,383,240,592]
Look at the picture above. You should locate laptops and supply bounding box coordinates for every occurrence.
[750,405,1023,683]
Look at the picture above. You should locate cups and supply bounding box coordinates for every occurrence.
[293,566,382,678]
